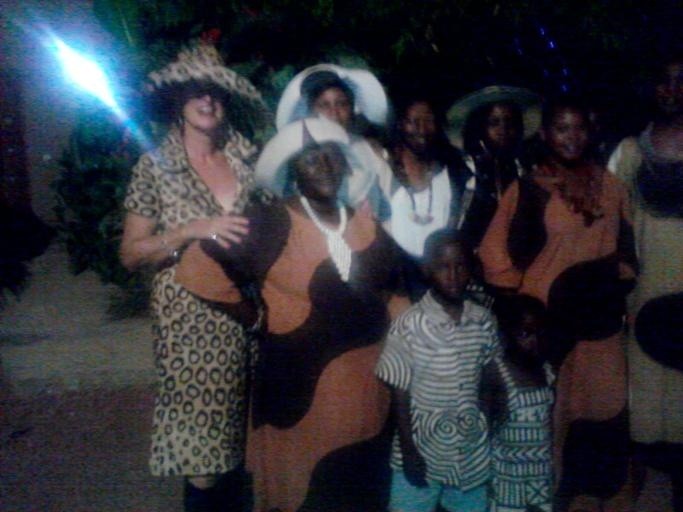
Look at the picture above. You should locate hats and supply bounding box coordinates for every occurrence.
[274,65,390,129]
[253,118,349,197]
[445,85,544,153]
[139,38,269,132]
